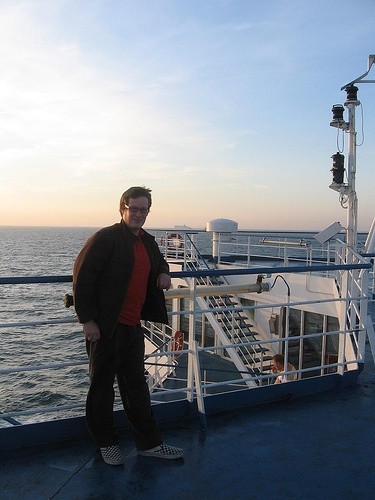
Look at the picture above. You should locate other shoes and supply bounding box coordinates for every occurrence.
[99,445,128,465]
[139,443,186,459]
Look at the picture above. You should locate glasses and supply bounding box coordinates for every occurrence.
[125,204,149,214]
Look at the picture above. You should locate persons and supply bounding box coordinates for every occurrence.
[72,187,184,465]
[272,354,297,384]
